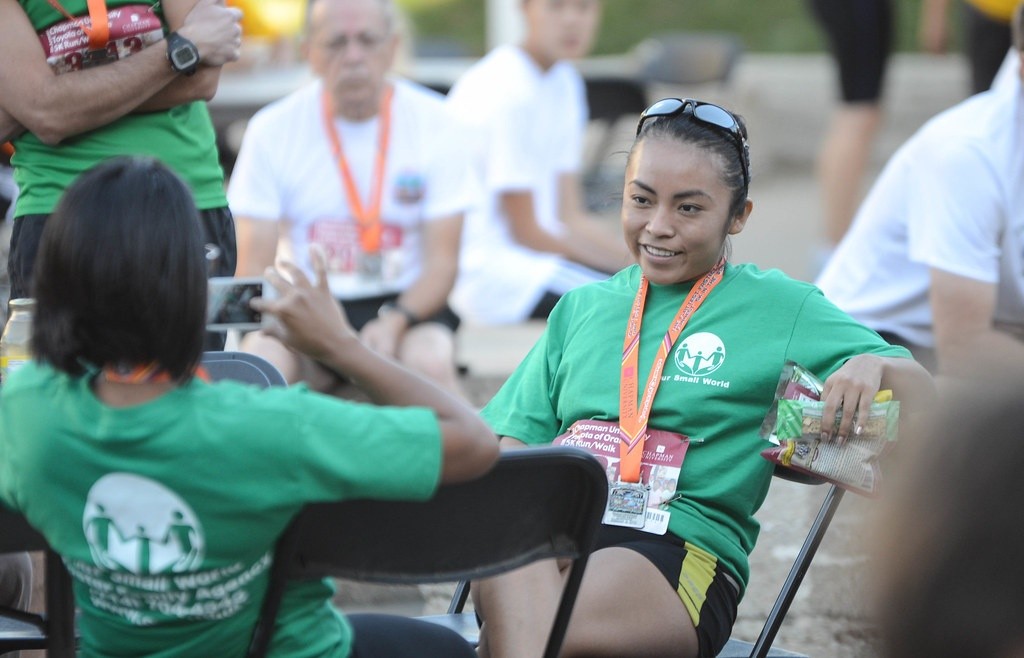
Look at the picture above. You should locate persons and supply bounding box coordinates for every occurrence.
[226,0,472,398]
[448,0,634,323]
[212,286,259,323]
[645,468,677,511]
[1,155,499,658]
[0,0,242,349]
[805,0,1024,658]
[474,97,932,658]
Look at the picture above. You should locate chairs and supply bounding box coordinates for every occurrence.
[236,448,608,658]
[586,76,648,206]
[448,437,845,658]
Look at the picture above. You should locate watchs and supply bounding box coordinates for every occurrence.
[167,32,200,77]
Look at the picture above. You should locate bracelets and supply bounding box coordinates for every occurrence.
[378,301,419,329]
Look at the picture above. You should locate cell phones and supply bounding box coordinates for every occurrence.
[207,275,278,331]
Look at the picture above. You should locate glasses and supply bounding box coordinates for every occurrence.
[635,96,750,194]
